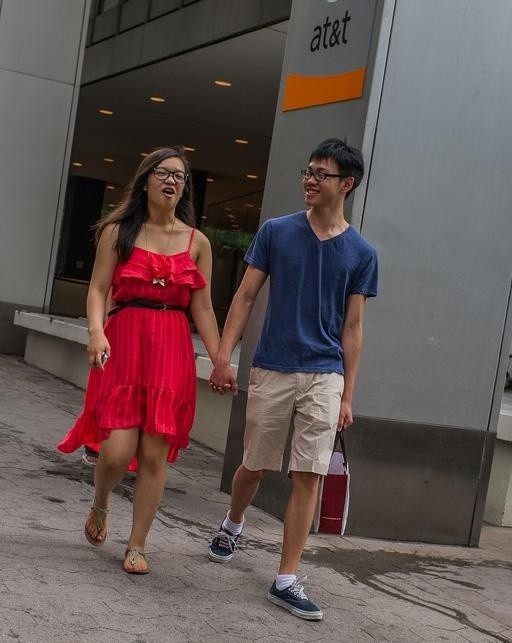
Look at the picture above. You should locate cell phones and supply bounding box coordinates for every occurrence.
[96,350,108,367]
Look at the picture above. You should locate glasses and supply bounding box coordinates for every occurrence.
[152,167,189,185]
[300,169,342,183]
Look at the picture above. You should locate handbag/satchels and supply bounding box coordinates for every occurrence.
[313,428,351,538]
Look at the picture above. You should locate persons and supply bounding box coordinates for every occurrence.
[205,135,378,623]
[55,147,238,575]
[80,285,113,473]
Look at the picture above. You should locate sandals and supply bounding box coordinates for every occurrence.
[84,496,109,548]
[123,544,149,576]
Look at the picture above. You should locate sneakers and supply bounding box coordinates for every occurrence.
[267,578,324,621]
[208,514,244,563]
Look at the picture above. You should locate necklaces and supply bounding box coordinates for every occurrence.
[143,217,179,258]
